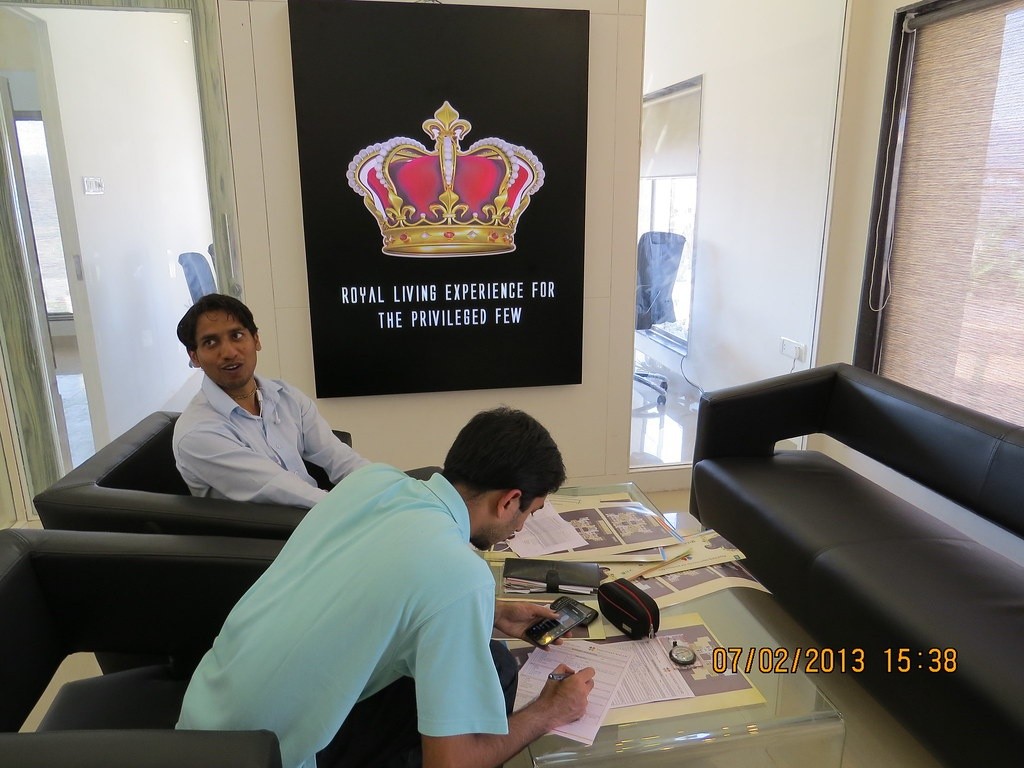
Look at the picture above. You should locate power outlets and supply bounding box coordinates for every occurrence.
[780,336,807,362]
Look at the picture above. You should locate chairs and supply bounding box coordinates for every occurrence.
[636,230,685,404]
[179,252,218,306]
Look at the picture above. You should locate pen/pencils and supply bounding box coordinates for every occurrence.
[548,672,571,680]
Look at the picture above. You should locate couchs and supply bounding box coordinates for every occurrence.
[0,527,289,768]
[36,410,353,676]
[688,362,1024,768]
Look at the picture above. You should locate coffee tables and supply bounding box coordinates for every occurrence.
[503,481,846,768]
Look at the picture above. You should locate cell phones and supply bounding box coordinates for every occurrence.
[525,602,586,645]
[550,596,598,626]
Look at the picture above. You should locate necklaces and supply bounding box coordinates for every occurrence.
[231,381,257,400]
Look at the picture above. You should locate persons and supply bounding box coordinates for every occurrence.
[173,404,595,768]
[174,293,373,510]
